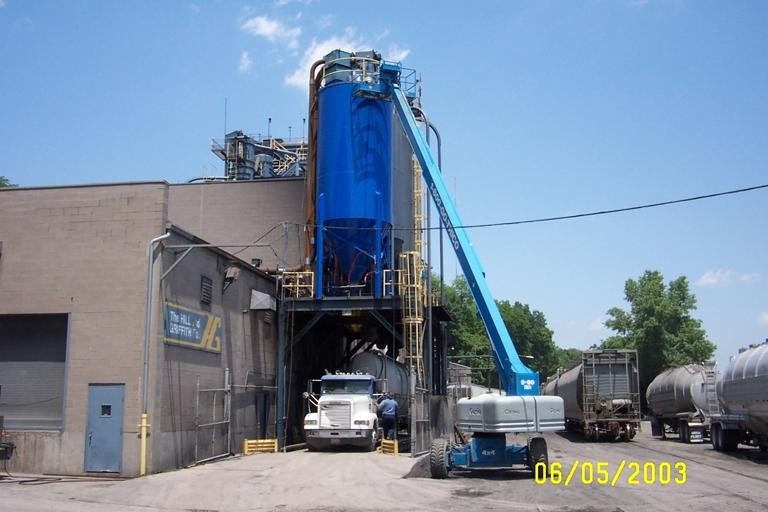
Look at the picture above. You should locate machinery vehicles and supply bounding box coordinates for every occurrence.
[392,83,565,479]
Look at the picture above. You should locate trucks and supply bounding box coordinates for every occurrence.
[646,339,768,451]
[540,349,641,443]
[302,352,409,452]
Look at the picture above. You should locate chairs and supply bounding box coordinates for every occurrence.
[332,388,369,393]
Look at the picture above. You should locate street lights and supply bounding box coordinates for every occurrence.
[138,232,171,477]
[519,355,534,359]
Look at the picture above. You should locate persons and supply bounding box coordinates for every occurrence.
[374,392,399,440]
